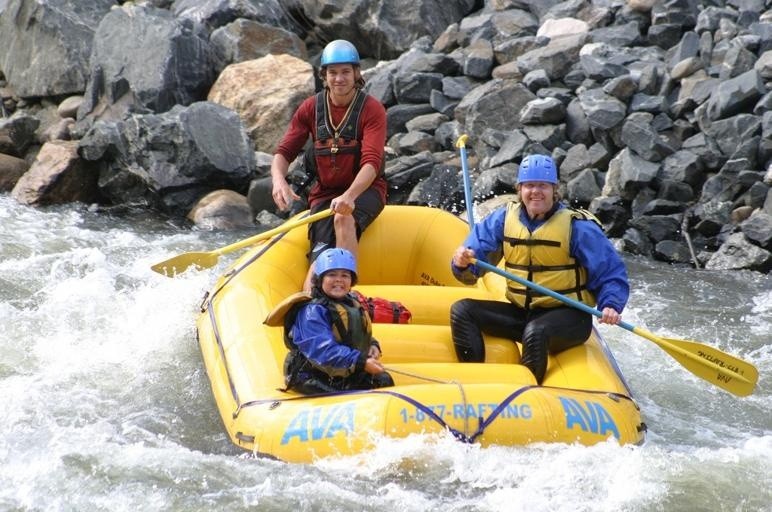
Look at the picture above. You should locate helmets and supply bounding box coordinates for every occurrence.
[315,248,357,277]
[321,40,359,65]
[517,155,558,186]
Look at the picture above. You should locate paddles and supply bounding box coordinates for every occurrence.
[150,208,334,276]
[469,256,759,398]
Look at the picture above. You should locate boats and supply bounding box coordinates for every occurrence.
[196,201,648,469]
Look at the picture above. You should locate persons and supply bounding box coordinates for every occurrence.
[282,248,393,396]
[270,39,387,294]
[450,153,630,388]
